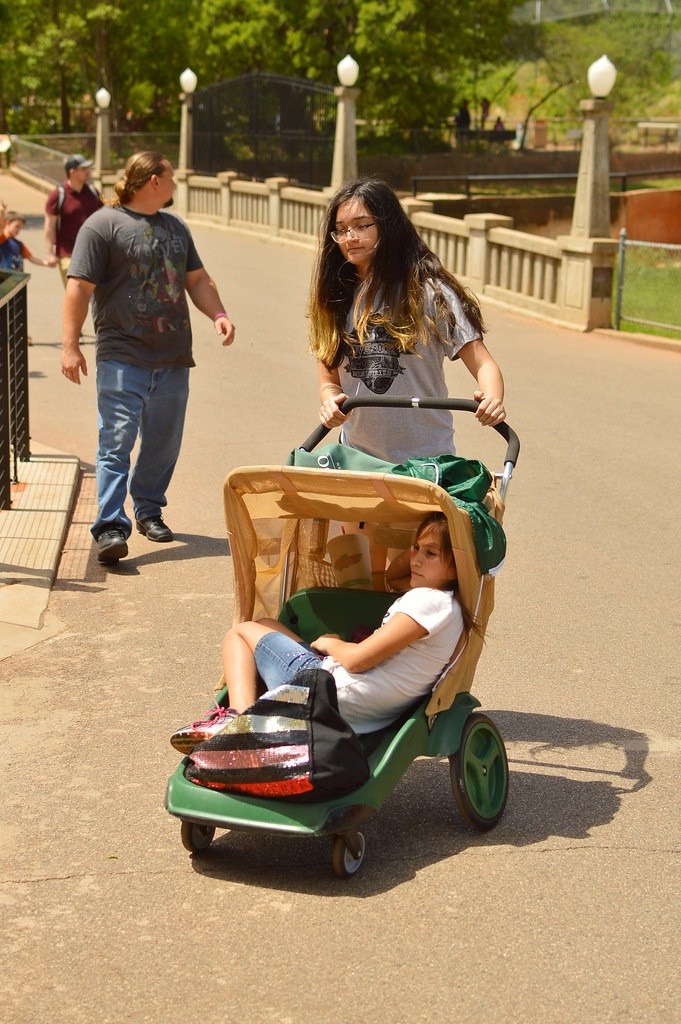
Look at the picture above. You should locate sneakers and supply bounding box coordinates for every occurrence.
[170,699,241,755]
[136,515,173,542]
[98,529,128,562]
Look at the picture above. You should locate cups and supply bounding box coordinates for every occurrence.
[327,534,374,592]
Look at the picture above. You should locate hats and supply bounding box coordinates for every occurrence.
[64,154,94,171]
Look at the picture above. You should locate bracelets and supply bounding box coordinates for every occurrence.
[215,312,228,320]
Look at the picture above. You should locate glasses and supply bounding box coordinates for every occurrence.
[331,222,374,243]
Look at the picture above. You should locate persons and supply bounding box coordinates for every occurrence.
[305,178,506,591]
[0,200,59,346]
[454,97,472,154]
[44,154,105,345]
[61,151,235,562]
[170,512,465,756]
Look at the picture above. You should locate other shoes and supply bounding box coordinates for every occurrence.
[78,337,83,343]
[28,338,32,345]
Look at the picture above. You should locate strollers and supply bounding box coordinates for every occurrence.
[165,394,522,878]
